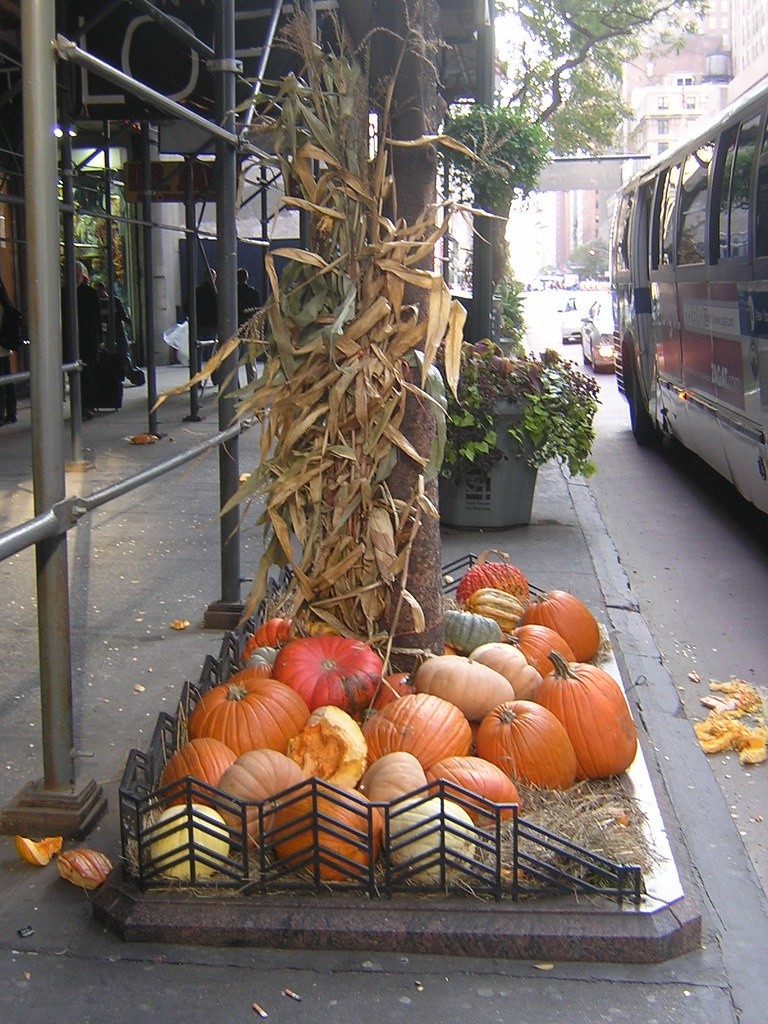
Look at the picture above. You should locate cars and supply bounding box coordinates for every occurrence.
[579,299,615,373]
[556,298,584,345]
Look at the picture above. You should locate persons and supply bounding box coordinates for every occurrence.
[62,261,133,417]
[237,267,261,388]
[195,269,217,390]
[0,277,18,426]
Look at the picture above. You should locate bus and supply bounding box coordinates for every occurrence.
[607,71,768,516]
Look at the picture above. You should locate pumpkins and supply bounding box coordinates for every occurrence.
[148,551,638,882]
[15,835,63,866]
[56,849,113,891]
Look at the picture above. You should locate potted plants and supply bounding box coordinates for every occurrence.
[431,339,599,528]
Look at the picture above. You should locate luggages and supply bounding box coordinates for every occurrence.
[88,344,126,412]
